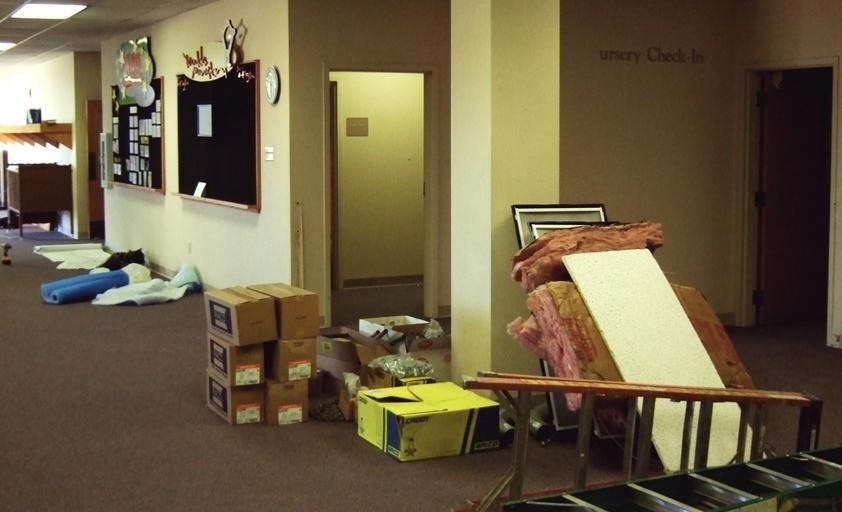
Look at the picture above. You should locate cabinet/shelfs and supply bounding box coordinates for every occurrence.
[6,165,73,238]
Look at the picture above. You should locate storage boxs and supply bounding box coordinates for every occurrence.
[355,381,501,464]
[203,284,430,425]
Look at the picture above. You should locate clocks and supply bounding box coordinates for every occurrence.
[266,67,280,103]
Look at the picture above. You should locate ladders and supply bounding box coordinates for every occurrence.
[463,371,822,512]
[499,447,842,512]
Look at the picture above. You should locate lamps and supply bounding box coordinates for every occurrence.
[0,42,16,51]
[11,4,88,21]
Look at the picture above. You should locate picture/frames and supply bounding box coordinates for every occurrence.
[512,204,608,439]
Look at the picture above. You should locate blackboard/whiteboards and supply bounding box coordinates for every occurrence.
[112,78,165,193]
[177,60,261,212]
